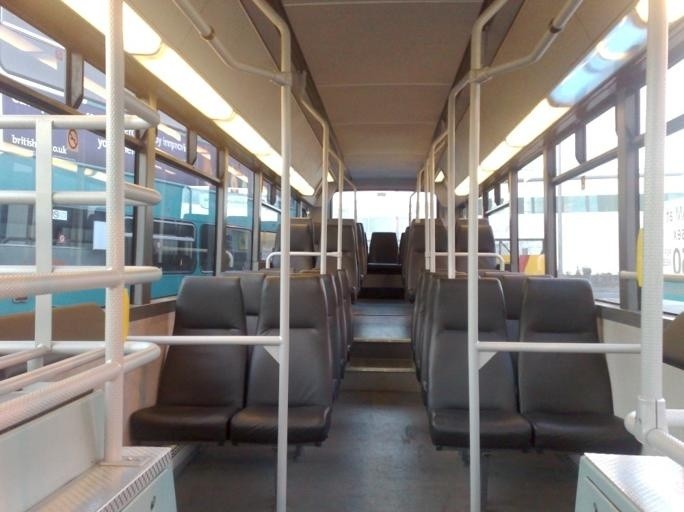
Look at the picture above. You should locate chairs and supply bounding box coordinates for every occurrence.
[128,219,368,464]
[368,231,402,274]
[399,215,643,465]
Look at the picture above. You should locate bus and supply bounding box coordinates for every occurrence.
[0,141,281,317]
